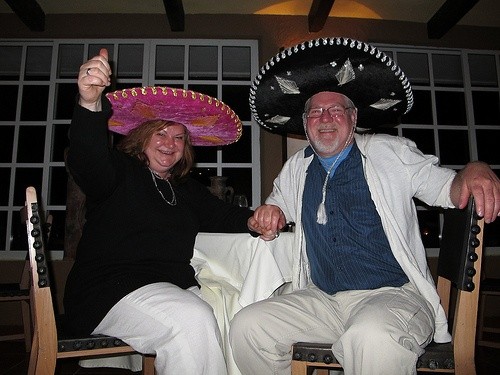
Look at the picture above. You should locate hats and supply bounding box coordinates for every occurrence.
[105,87,242,147]
[249,37,415,136]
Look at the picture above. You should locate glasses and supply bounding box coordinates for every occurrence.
[305,106,354,118]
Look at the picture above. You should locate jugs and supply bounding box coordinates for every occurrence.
[207,176,233,208]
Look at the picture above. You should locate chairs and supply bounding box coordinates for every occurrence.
[20,187,156,375]
[291,194,484,375]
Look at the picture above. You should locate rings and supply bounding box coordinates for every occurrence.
[86,68,91,75]
[274,231,279,238]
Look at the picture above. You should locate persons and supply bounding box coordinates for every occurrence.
[230,85,500,375]
[63,48,279,375]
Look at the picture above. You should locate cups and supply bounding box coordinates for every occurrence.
[233,195,248,209]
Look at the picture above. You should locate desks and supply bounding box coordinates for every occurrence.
[191,233,296,375]
